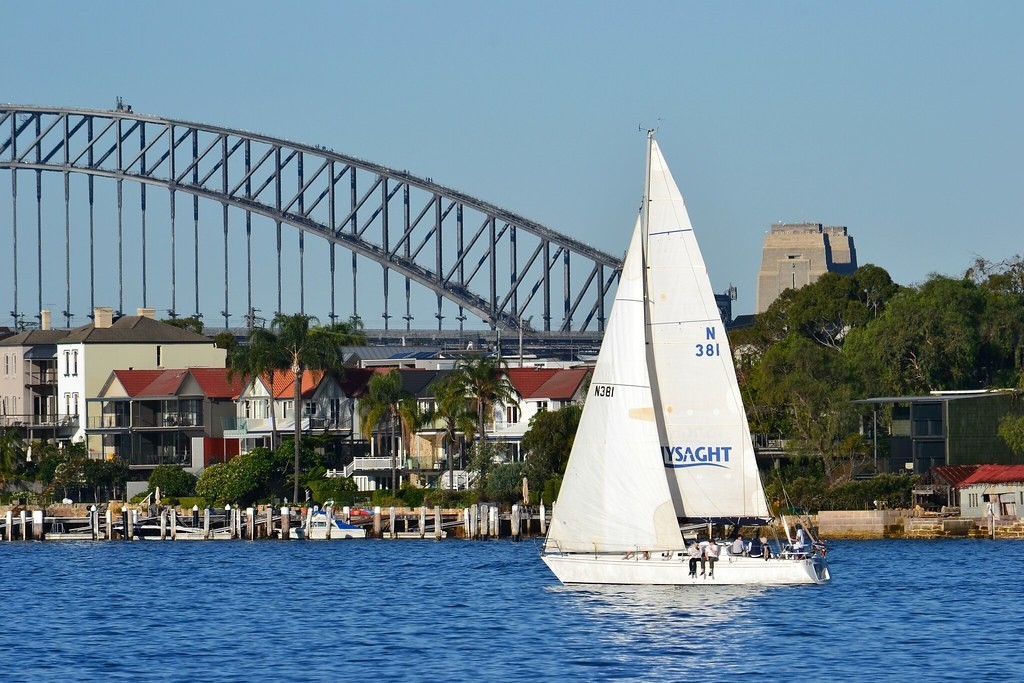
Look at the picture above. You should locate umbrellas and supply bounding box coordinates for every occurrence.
[523,478,529,512]
[155,487,161,516]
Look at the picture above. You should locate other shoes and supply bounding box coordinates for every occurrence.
[689,572,692,575]
[693,572,696,575]
[709,572,712,576]
[701,572,705,575]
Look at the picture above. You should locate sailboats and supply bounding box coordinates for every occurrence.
[532,127,830,586]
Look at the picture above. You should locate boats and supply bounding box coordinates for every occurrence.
[381,528,447,540]
[275,512,368,539]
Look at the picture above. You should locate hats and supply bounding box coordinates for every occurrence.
[708,539,715,542]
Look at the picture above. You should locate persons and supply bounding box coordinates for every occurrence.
[721,543,733,555]
[790,524,804,558]
[760,537,770,556]
[167,414,177,425]
[688,539,702,575]
[733,534,744,554]
[747,535,761,558]
[700,539,718,576]
